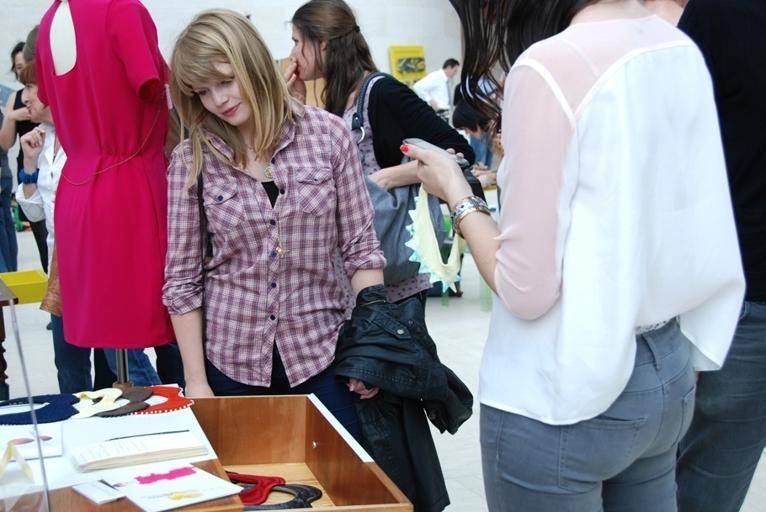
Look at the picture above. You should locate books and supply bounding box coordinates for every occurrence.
[63,428,208,473]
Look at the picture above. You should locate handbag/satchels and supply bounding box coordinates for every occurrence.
[359,142,447,285]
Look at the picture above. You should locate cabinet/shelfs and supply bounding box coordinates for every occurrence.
[0,383,414,512]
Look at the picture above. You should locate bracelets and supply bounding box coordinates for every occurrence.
[450,195,491,238]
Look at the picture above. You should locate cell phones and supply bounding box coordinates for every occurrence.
[402,137,470,168]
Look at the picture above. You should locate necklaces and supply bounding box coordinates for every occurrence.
[246,144,273,178]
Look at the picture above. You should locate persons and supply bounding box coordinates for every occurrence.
[163,10,385,444]
[643,0,766,512]
[2,24,183,400]
[283,0,475,310]
[32,1,173,349]
[410,59,505,172]
[400,0,746,512]
